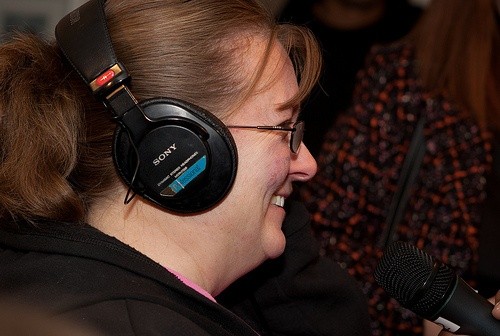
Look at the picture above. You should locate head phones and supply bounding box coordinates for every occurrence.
[55,0,238,214]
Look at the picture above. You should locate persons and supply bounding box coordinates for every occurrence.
[0,0,371,336]
[272,0,431,166]
[292,1,500,336]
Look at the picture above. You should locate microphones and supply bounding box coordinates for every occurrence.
[373,241,500,336]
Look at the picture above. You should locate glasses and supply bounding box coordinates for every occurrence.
[227,119,304,154]
